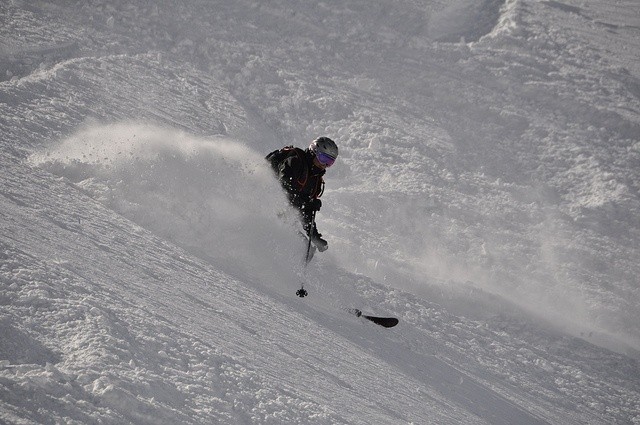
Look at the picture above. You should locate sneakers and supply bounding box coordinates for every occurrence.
[311,232,329,252]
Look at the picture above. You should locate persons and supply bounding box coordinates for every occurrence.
[265,137,338,267]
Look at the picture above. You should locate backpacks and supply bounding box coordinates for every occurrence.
[265,145,325,205]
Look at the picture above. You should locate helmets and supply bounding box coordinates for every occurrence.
[308,137,338,159]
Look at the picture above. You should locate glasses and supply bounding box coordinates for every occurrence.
[311,142,336,167]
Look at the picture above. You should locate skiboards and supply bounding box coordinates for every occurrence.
[344,309,398,328]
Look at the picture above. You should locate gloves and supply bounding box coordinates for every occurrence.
[301,197,321,215]
